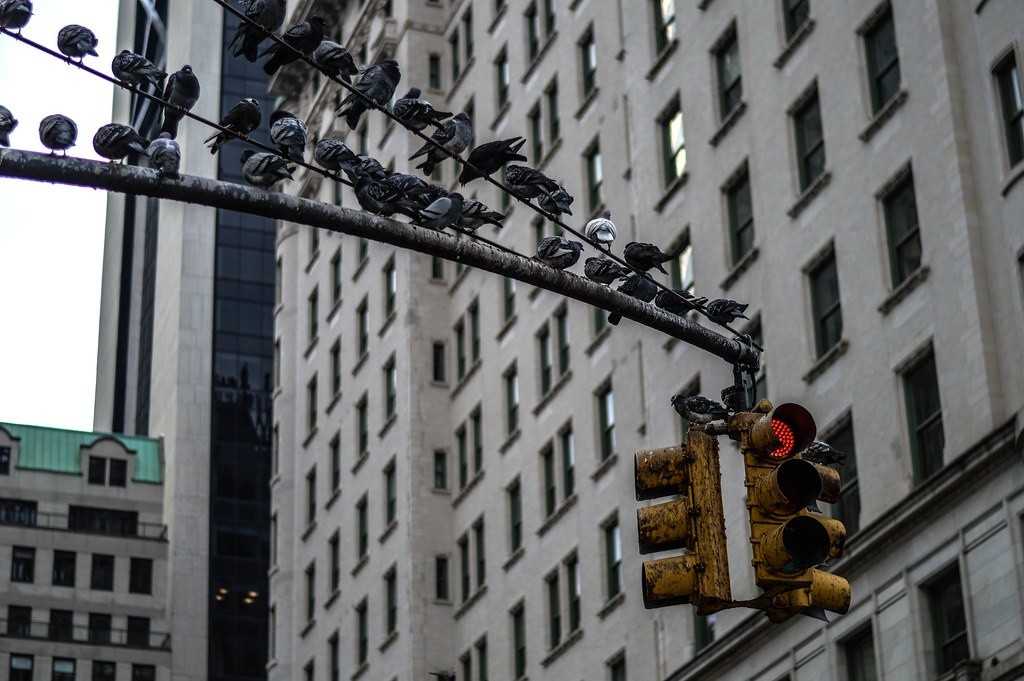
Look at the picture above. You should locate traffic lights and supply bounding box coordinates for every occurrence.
[770,453,851,625]
[730,401,831,589]
[634,431,733,617]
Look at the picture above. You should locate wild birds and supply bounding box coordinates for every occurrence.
[797,439,849,463]
[670,394,731,424]
[0,1,753,327]
